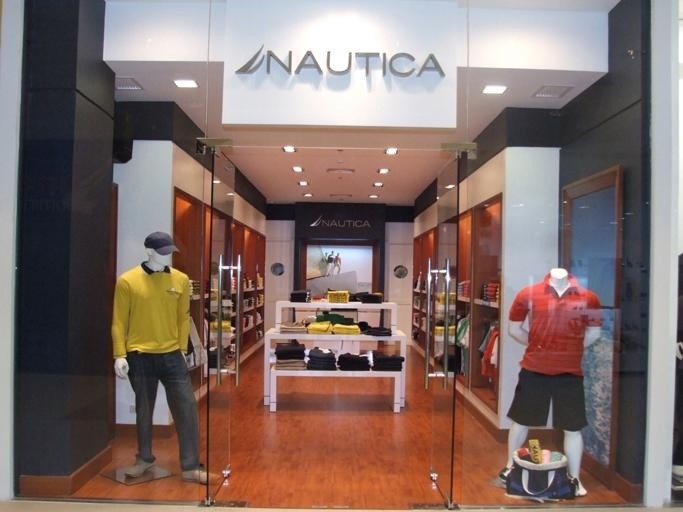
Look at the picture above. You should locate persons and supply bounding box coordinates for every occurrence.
[324,250,335,277]
[317,251,328,278]
[110,230,215,484]
[506,268,602,499]
[333,252,341,274]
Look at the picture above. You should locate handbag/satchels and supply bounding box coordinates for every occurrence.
[506,449,574,504]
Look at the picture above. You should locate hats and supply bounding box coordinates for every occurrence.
[144,232,179,255]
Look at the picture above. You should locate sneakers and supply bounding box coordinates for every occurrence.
[180,469,216,484]
[498,466,510,481]
[123,458,155,477]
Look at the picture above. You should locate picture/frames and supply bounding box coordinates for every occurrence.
[561,164,625,491]
[299,239,379,293]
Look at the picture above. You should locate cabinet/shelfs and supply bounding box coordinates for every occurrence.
[173,188,264,393]
[413,193,501,413]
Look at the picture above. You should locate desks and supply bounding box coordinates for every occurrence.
[263,300,407,413]
